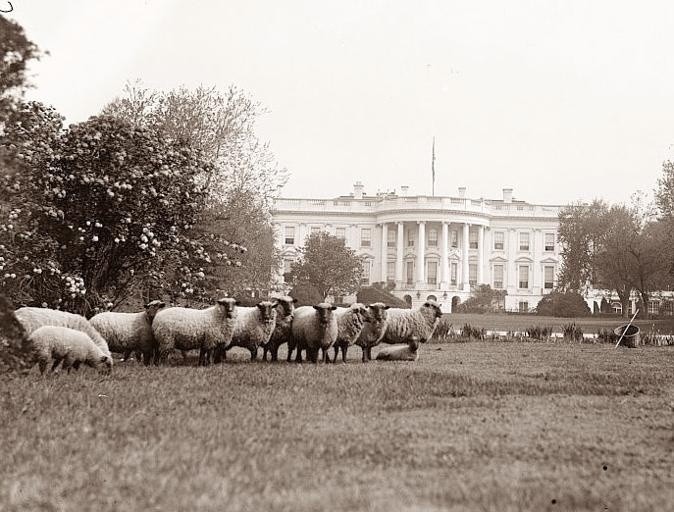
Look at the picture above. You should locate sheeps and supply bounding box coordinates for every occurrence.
[378,299,445,345]
[376,334,421,361]
[88,299,166,367]
[324,301,370,365]
[260,296,297,364]
[227,299,281,364]
[11,305,115,380]
[154,294,241,367]
[353,301,391,364]
[287,301,338,365]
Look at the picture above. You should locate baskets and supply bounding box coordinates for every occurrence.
[614,323,641,348]
